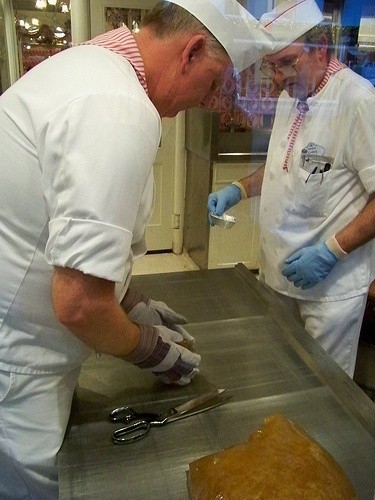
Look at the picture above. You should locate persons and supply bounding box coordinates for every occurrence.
[0,0,276,500]
[206,0,375,379]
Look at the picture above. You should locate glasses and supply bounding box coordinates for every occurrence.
[259,45,304,81]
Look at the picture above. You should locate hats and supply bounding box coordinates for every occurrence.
[164,0,280,77]
[258,0,325,58]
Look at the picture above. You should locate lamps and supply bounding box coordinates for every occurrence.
[20,0,69,38]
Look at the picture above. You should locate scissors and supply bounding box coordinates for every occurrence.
[107,386,234,445]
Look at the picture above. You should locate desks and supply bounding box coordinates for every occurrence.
[56,263,375,500]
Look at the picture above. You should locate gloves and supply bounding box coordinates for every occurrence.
[207,181,248,227]
[119,320,201,386]
[281,234,349,291]
[119,287,198,353]
